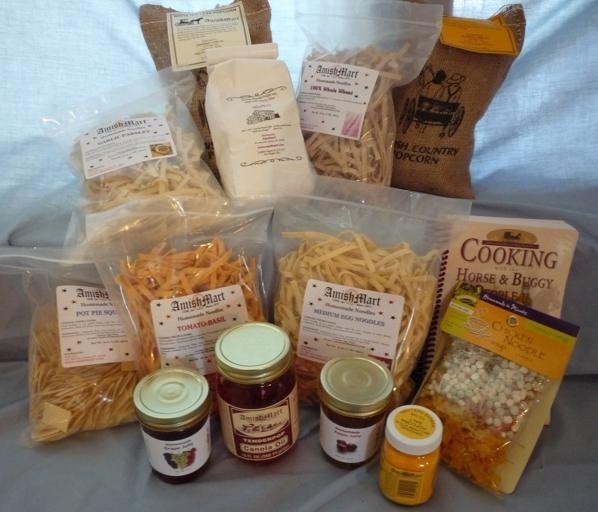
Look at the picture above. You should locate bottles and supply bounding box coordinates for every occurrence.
[133,366,211,484]
[319,354,393,470]
[214,322,299,464]
[377,405,443,506]
